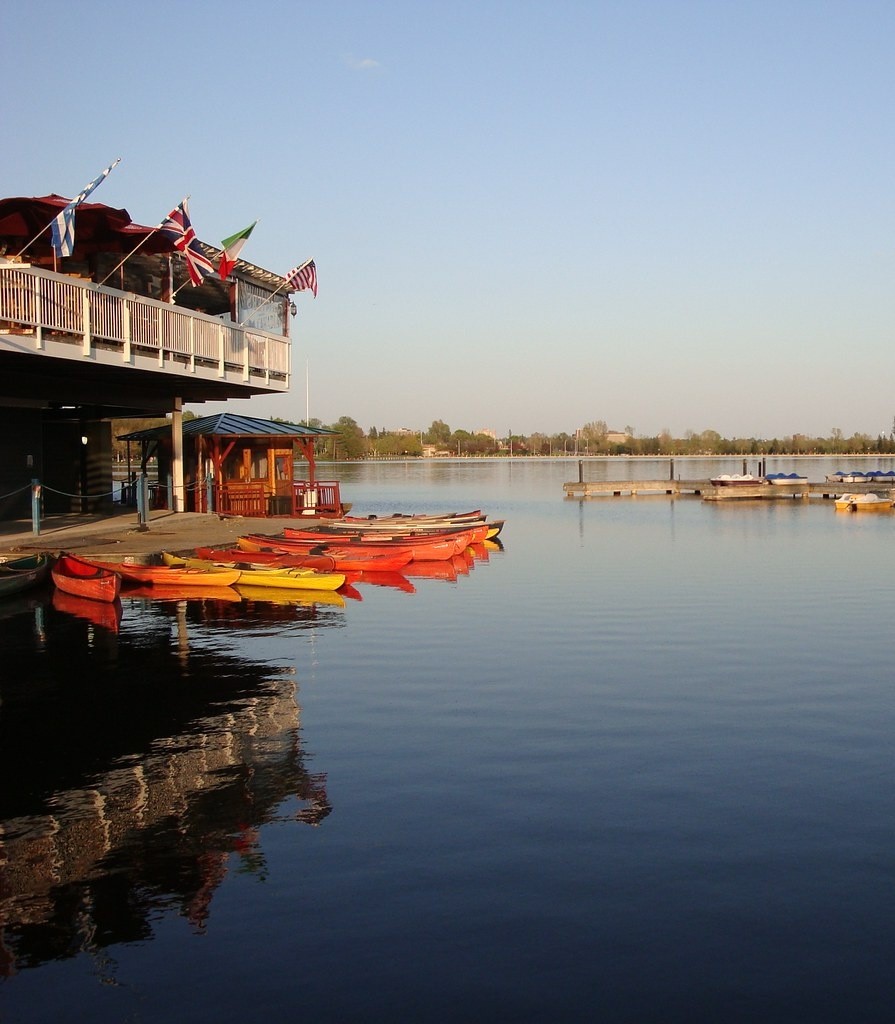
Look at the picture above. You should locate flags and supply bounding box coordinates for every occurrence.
[218,221,257,280]
[284,259,317,299]
[53,162,118,257]
[155,199,214,287]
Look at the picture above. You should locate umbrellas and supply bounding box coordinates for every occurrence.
[0,194,181,290]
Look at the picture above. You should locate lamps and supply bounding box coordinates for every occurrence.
[288,298,297,316]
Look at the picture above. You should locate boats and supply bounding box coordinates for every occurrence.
[482,538,501,553]
[335,583,363,602]
[709,471,764,489]
[113,582,242,605]
[823,470,895,483]
[445,554,469,577]
[489,535,505,553]
[397,560,458,582]
[470,542,489,561]
[90,559,245,587]
[334,570,416,594]
[228,582,348,610]
[0,552,56,596]
[50,586,123,639]
[764,473,809,486]
[51,549,124,604]
[190,509,506,573]
[465,544,476,560]
[833,492,894,512]
[161,550,348,592]
[457,549,476,570]
